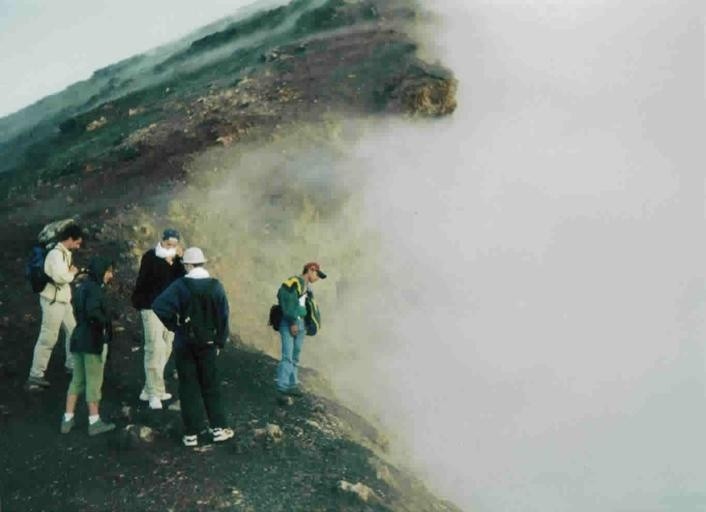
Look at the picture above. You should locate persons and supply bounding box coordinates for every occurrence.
[275,261,328,393]
[60,254,118,437]
[131,227,188,411]
[151,246,235,448]
[28,223,86,386]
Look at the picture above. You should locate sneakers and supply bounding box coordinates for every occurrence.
[29,375,50,386]
[88,417,116,436]
[183,435,198,446]
[149,396,162,409]
[213,426,234,442]
[61,415,75,433]
[67,368,74,374]
[139,390,172,401]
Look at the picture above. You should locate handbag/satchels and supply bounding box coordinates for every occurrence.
[268,305,285,330]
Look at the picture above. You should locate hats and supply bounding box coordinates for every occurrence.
[305,262,327,279]
[163,229,179,241]
[180,247,208,264]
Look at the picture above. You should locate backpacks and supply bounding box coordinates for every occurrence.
[26,242,59,292]
